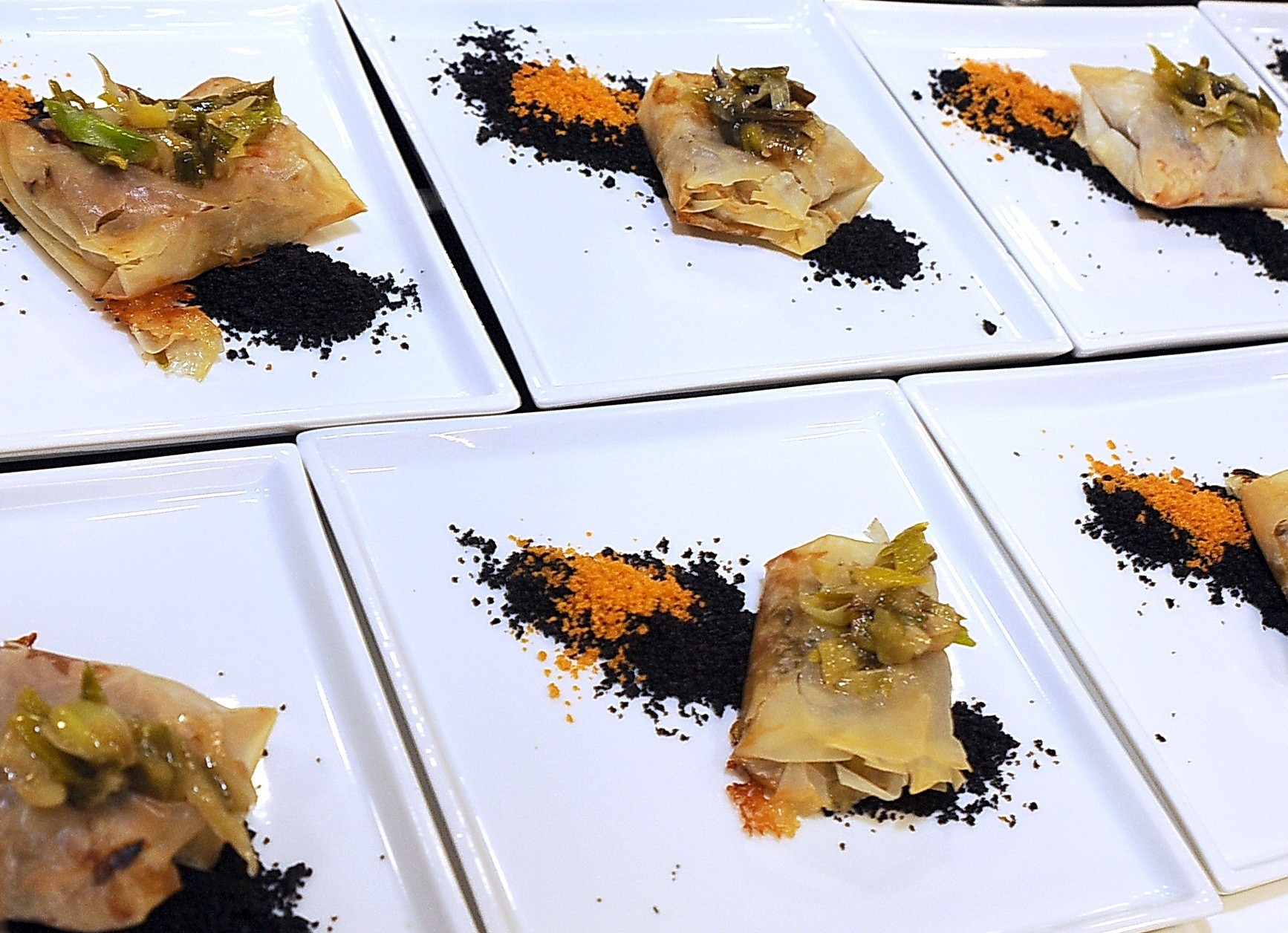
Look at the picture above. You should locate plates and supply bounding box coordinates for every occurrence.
[2,5,524,462]
[297,376,1229,933]
[827,0,1288,359]
[2,445,481,933]
[896,342,1288,896]
[339,1,1076,411]
[1199,0,1287,108]
